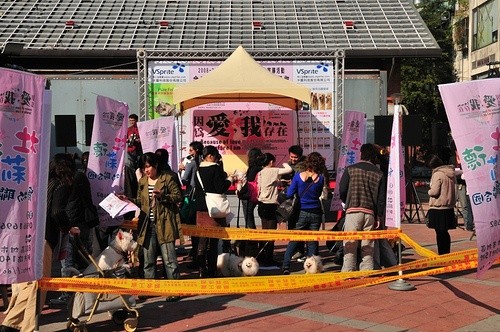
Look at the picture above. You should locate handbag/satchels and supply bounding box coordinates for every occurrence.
[205,193,231,218]
[326,212,345,255]
[238,181,248,200]
[276,194,297,222]
[179,186,196,225]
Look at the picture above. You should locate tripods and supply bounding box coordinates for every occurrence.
[404,146,426,224]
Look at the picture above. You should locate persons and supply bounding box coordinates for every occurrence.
[43,151,139,306]
[235,144,330,275]
[135,140,239,304]
[338,143,398,277]
[449,141,474,230]
[424,154,456,256]
[127,113,143,171]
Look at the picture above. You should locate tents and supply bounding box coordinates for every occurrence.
[173,45,313,240]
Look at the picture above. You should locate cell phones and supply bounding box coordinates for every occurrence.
[153,191,160,194]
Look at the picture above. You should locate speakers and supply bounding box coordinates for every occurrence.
[374,115,393,146]
[85,114,94,146]
[55,115,77,148]
[402,114,423,146]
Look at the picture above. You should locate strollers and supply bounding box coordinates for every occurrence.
[66,233,140,332]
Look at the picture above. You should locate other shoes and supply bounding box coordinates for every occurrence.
[291,250,305,260]
[283,268,290,275]
[138,296,153,299]
[50,298,66,305]
[166,296,180,302]
[183,256,193,260]
[178,248,184,255]
[297,256,307,262]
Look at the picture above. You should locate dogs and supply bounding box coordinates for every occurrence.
[303,255,322,275]
[217,253,259,277]
[61,229,139,276]
